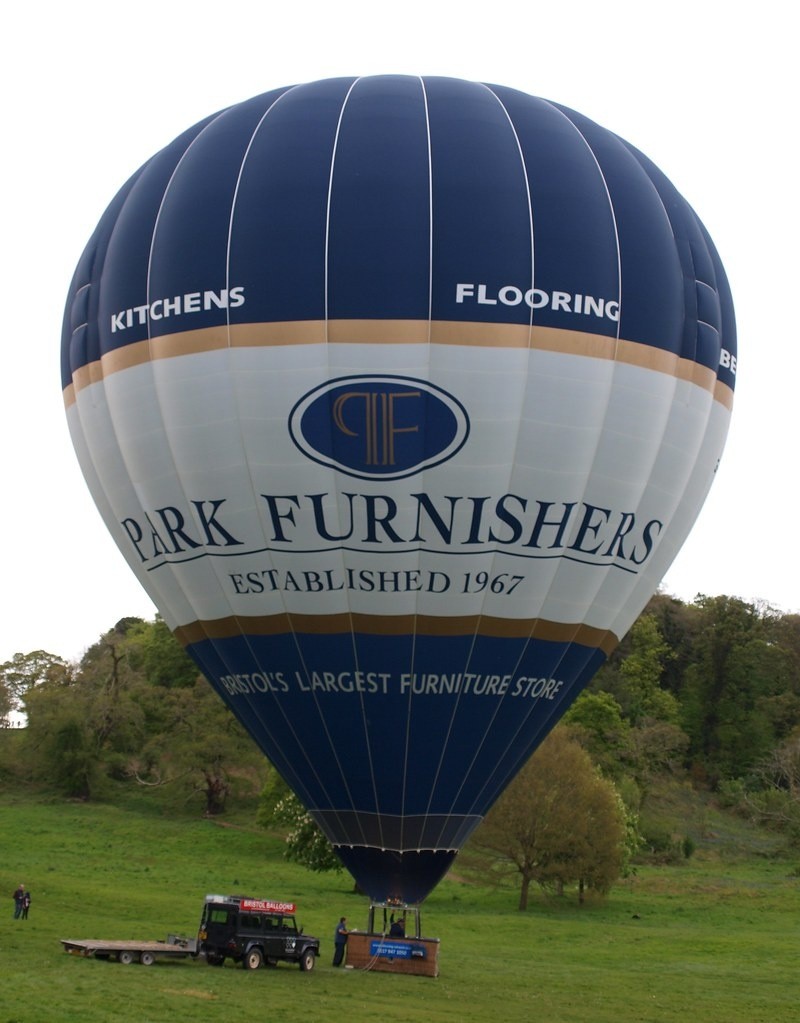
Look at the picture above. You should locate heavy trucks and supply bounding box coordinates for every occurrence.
[60,894,266,966]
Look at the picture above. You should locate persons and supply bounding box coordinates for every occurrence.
[22,892,31,920]
[12,884,24,920]
[390,910,404,937]
[333,917,350,966]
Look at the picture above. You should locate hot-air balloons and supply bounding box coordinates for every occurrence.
[59,69,739,978]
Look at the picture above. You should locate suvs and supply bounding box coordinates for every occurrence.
[199,901,321,972]
